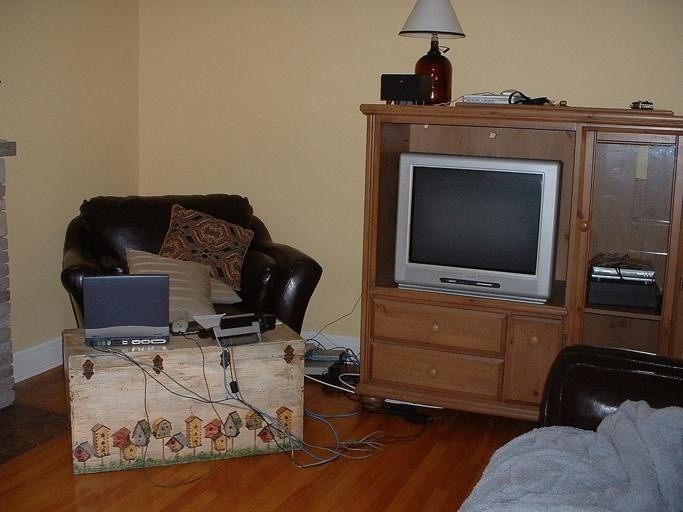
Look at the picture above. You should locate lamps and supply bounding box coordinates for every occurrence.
[399,0,465,106]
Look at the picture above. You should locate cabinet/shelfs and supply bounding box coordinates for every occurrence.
[353,103,683,422]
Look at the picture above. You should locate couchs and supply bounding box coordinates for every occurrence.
[455,345,683,511]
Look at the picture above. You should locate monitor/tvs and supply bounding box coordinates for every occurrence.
[394,152,561,305]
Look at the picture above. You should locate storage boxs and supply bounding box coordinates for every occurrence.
[61,320,307,477]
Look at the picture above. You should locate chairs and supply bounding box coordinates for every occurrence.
[60,193,323,336]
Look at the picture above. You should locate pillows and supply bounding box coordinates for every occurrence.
[123,247,216,324]
[159,203,255,292]
[208,276,243,305]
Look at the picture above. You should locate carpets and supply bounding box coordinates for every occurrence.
[0,404,68,465]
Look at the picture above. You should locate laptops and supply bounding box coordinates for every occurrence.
[82,274,170,346]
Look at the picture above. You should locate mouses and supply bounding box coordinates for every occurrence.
[172,319,189,333]
